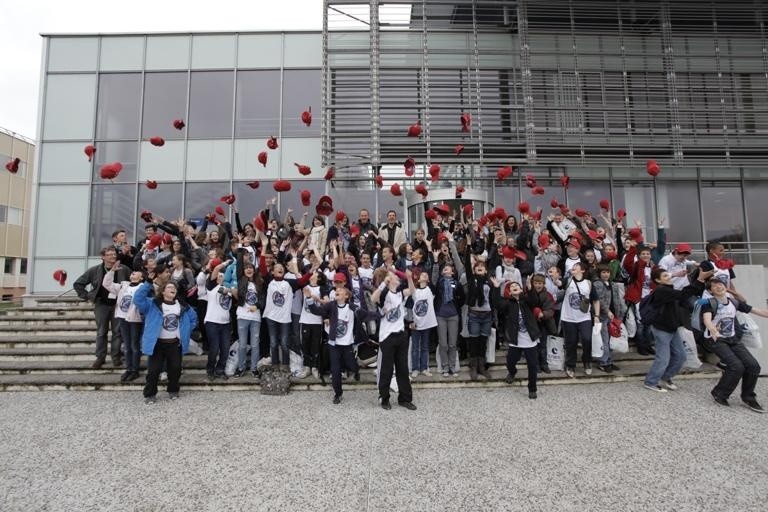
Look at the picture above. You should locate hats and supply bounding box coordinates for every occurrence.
[587,228,597,240]
[7,157,21,173]
[675,243,693,254]
[407,124,423,137]
[266,135,278,149]
[608,318,621,337]
[332,272,347,284]
[617,209,627,219]
[215,163,336,216]
[145,180,157,189]
[86,146,96,162]
[173,119,185,131]
[709,250,735,268]
[478,207,514,259]
[151,137,164,147]
[254,209,305,245]
[629,229,643,242]
[258,151,268,167]
[53,271,68,285]
[537,233,549,250]
[504,282,512,297]
[599,199,610,211]
[460,114,472,132]
[301,106,312,126]
[140,208,171,250]
[566,237,581,249]
[566,231,583,240]
[100,163,122,180]
[496,167,586,214]
[646,158,661,176]
[375,159,473,220]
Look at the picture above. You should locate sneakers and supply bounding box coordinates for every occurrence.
[208,366,260,382]
[609,364,619,370]
[660,377,678,390]
[650,345,656,354]
[113,359,122,366]
[506,369,517,384]
[598,365,612,373]
[637,347,649,355]
[333,393,342,404]
[311,367,320,379]
[144,392,157,405]
[737,393,765,414]
[710,387,732,409]
[378,395,391,410]
[583,361,593,375]
[528,389,537,399]
[295,365,311,379]
[412,369,459,378]
[120,371,127,383]
[168,391,180,401]
[566,365,575,378]
[398,400,416,410]
[127,370,139,382]
[92,357,105,369]
[643,379,668,393]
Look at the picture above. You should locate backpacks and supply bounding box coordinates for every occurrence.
[691,297,739,331]
[640,294,662,323]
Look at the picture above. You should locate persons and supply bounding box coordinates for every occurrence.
[73,197,767,413]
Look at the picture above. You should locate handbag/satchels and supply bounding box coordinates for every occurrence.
[592,322,604,358]
[737,312,764,350]
[677,326,703,370]
[485,325,496,364]
[224,338,252,376]
[546,334,566,371]
[580,295,590,313]
[624,307,637,338]
[288,346,305,374]
[259,365,292,396]
[353,339,381,367]
[610,325,630,353]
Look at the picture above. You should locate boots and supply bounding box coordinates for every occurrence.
[469,358,487,381]
[479,355,492,379]
